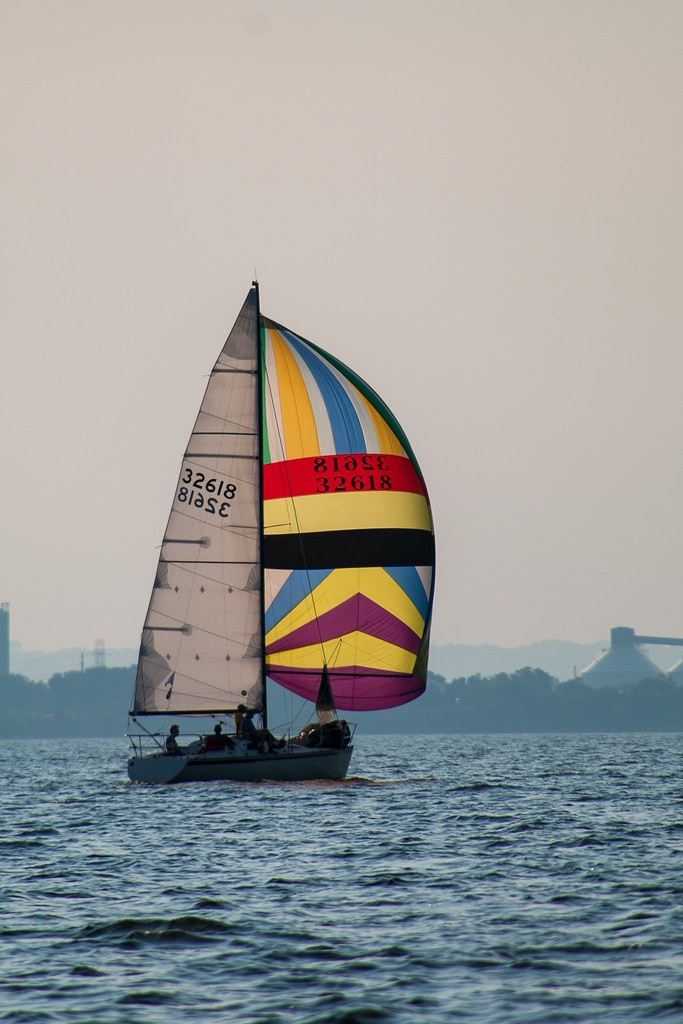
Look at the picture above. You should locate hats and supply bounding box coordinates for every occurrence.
[238,704,248,709]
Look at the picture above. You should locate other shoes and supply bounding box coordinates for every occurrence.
[258,750,268,754]
[268,749,277,754]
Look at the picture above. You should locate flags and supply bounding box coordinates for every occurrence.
[316,664,336,727]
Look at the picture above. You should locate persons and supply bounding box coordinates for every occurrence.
[235,704,280,754]
[201,725,237,753]
[166,724,182,756]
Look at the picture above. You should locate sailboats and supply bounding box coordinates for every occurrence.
[124,279,438,783]
